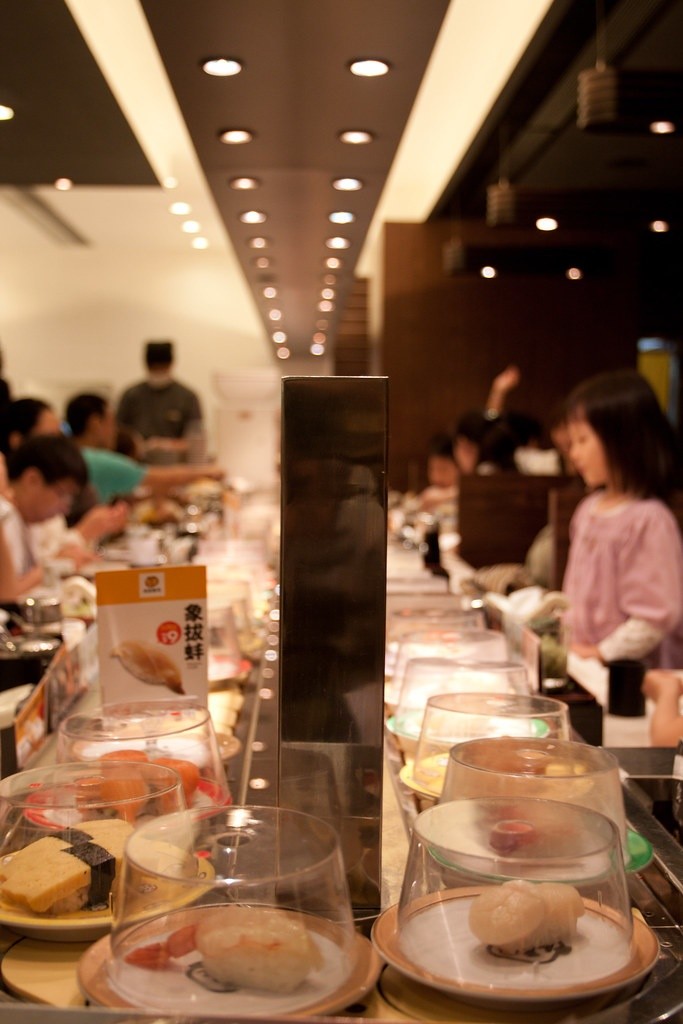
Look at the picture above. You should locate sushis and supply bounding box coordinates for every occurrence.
[108,643,185,694]
[1,742,585,995]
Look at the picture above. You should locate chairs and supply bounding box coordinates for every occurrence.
[456,471,606,598]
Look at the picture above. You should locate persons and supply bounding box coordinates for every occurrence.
[1,336,221,603]
[415,361,607,598]
[561,366,682,674]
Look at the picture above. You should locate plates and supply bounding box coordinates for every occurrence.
[23,776,234,833]
[0,838,214,940]
[370,885,661,1011]
[426,827,654,883]
[71,733,242,769]
[399,753,596,803]
[387,712,551,746]
[76,906,384,1017]
[208,655,252,691]
